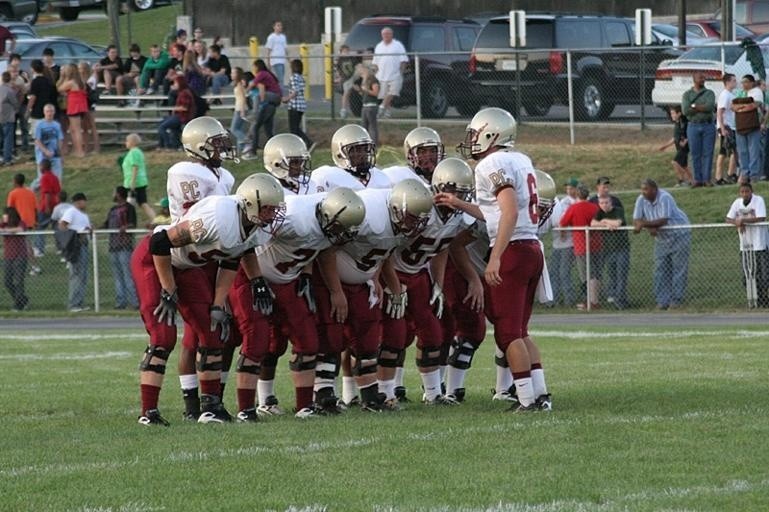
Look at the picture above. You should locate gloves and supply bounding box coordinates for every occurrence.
[210,305,231,343]
[153,287,179,326]
[250,277,276,316]
[385,285,408,319]
[429,282,444,319]
[298,273,317,313]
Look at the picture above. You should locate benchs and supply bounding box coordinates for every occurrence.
[17,82,259,149]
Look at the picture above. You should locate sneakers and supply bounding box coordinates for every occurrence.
[138,408,170,427]
[294,386,411,418]
[674,173,738,189]
[183,396,285,423]
[491,384,552,411]
[421,382,466,406]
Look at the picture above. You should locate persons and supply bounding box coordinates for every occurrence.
[100,186,143,309]
[726,182,769,306]
[220,59,317,159]
[123,135,171,226]
[131,173,287,427]
[30,106,62,191]
[552,177,629,310]
[159,27,232,150]
[169,115,556,423]
[433,109,551,412]
[634,179,691,310]
[659,71,768,186]
[337,27,408,144]
[1,25,170,165]
[0,159,94,310]
[266,20,291,95]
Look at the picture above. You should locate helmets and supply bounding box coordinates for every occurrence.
[182,116,234,167]
[389,106,556,236]
[237,123,376,245]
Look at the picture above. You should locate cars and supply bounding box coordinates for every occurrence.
[0,0,175,27]
[651,42,769,120]
[0,21,116,83]
[651,16,769,50]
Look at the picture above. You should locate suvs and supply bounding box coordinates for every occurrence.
[333,14,554,121]
[469,13,689,122]
